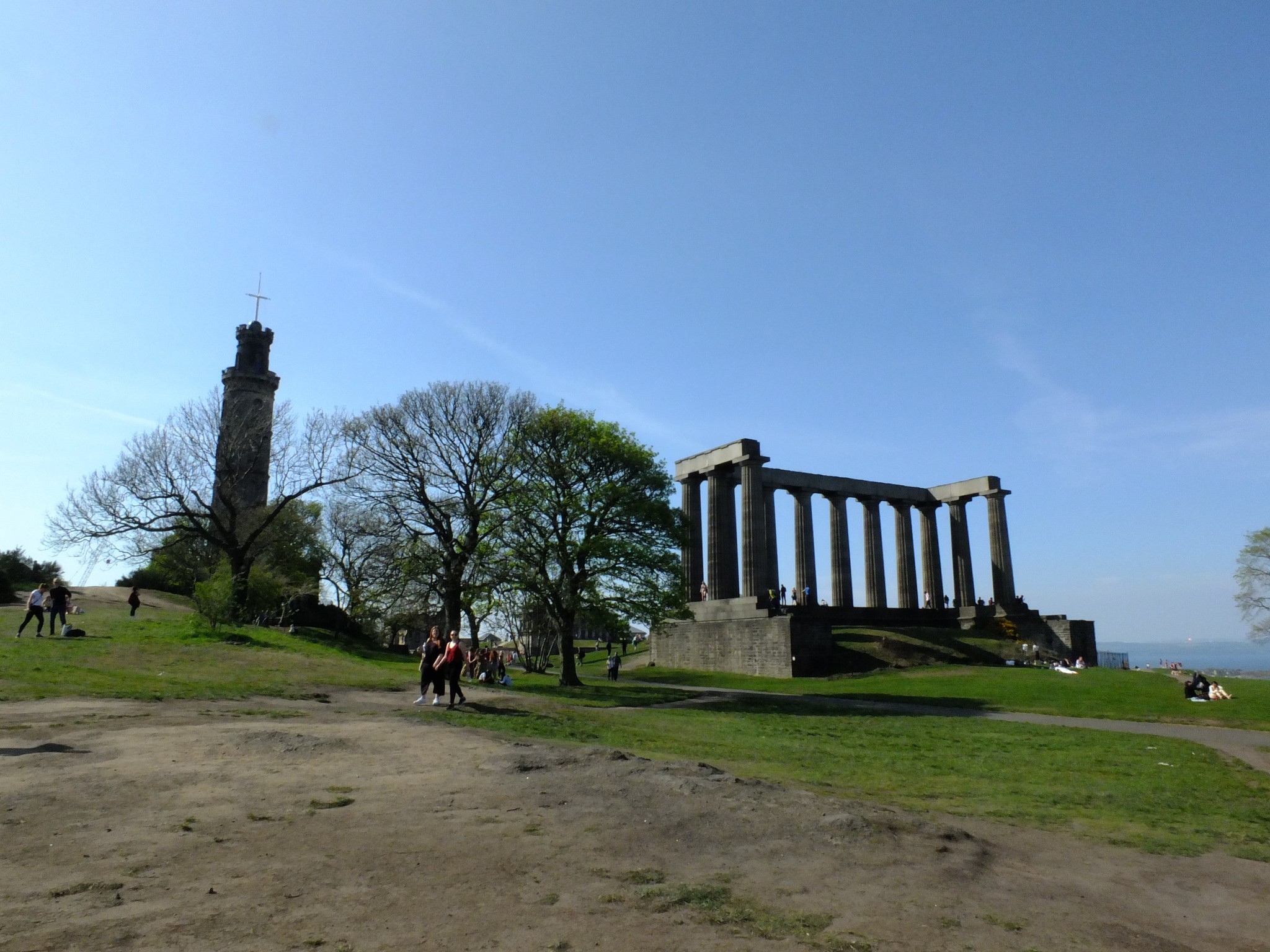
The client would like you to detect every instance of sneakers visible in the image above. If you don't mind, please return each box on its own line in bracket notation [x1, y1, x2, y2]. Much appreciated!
[413, 695, 427, 704]
[433, 697, 439, 705]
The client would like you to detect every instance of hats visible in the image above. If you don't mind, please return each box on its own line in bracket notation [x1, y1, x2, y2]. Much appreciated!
[480, 649, 484, 652]
[492, 648, 495, 651]
[133, 587, 138, 591]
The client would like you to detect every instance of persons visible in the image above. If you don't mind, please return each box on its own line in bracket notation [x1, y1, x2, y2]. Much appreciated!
[1135, 658, 1231, 702]
[128, 587, 140, 616]
[1021, 641, 1086, 675]
[944, 594, 1024, 608]
[924, 591, 932, 608]
[16, 577, 71, 638]
[412, 626, 517, 710]
[767, 584, 828, 606]
[700, 581, 707, 601]
[577, 634, 639, 682]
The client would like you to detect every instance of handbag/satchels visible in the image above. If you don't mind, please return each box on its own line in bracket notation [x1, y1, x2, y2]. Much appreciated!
[61, 623, 73, 635]
[577, 651, 585, 659]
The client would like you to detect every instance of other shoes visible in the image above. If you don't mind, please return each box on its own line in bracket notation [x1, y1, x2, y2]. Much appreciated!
[447, 703, 455, 710]
[16, 633, 20, 638]
[1228, 694, 1232, 699]
[48, 632, 56, 636]
[1221, 695, 1223, 699]
[458, 696, 466, 705]
[36, 634, 44, 638]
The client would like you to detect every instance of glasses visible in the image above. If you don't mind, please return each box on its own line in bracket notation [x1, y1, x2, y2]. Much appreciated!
[54, 582, 59, 583]
[451, 634, 458, 636]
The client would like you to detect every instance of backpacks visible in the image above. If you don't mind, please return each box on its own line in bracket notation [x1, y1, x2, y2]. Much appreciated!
[71, 606, 79, 614]
[1202, 691, 1210, 700]
[478, 671, 489, 683]
[1061, 658, 1071, 667]
[64, 629, 86, 637]
